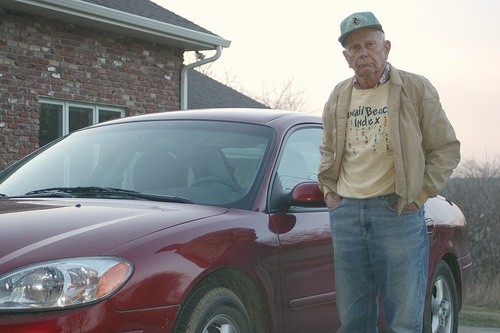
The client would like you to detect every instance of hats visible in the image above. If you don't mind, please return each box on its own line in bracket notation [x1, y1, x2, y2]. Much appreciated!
[338, 11, 382, 43]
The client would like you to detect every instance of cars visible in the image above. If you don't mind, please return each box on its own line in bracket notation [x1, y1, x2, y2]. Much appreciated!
[0, 107, 472, 333]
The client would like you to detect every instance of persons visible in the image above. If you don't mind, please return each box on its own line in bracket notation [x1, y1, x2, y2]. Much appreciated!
[318, 11, 461, 333]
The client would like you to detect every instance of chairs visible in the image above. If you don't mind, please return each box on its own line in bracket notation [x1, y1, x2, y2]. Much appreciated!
[257, 149, 310, 208]
